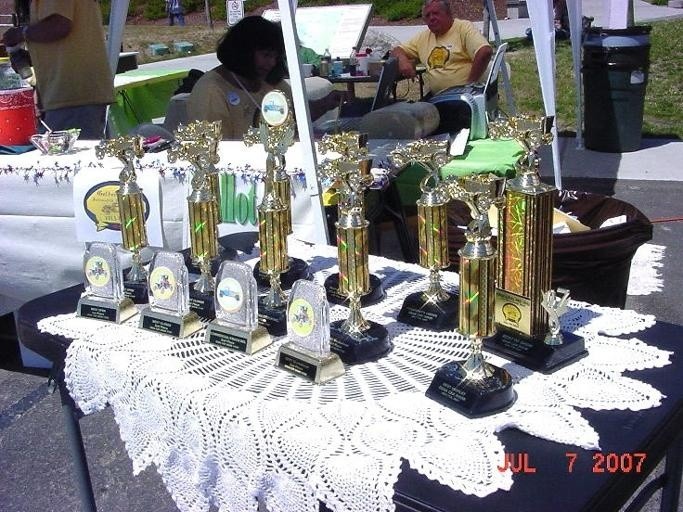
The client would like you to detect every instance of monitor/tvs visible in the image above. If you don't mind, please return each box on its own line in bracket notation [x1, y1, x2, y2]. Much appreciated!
[338, 58, 399, 118]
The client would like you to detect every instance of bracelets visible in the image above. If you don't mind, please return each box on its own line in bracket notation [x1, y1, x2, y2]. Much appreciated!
[21, 25, 29, 41]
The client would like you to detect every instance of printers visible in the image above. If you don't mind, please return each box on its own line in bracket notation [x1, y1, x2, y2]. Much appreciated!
[427, 42, 509, 141]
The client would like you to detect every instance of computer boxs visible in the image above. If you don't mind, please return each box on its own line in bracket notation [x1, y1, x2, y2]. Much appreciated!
[583, 26, 651, 154]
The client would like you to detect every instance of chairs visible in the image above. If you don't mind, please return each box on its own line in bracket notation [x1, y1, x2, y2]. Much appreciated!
[472, 43, 509, 139]
[312, 57, 399, 140]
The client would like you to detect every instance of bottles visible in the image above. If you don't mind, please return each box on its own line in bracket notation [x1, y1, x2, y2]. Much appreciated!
[333, 58, 343, 73]
[323, 48, 330, 63]
[348, 47, 357, 75]
[320, 60, 329, 77]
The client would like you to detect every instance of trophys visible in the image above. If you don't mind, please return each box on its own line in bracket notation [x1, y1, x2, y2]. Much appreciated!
[386, 137, 460, 332]
[94, 134, 157, 305]
[138, 250, 204, 339]
[204, 259, 273, 354]
[242, 89, 314, 335]
[424, 171, 518, 420]
[315, 129, 397, 363]
[166, 118, 224, 321]
[76, 240, 138, 324]
[274, 278, 346, 386]
[480, 108, 590, 375]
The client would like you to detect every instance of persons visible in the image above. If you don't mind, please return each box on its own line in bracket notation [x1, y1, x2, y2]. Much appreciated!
[186, 15, 292, 142]
[2, 0, 118, 141]
[390, 0, 494, 100]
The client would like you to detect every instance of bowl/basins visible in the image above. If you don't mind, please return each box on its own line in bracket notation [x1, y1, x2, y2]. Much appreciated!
[30, 132, 79, 154]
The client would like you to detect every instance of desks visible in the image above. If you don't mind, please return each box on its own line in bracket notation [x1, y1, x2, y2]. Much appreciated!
[0, 139, 415, 367]
[112, 53, 221, 125]
[17, 231, 683, 511]
[319, 66, 425, 102]
